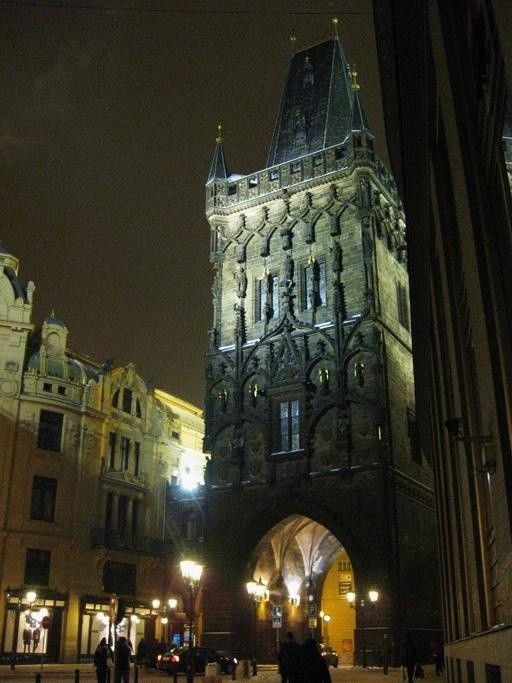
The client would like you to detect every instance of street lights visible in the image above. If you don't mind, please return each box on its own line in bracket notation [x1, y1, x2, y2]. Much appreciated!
[346, 587, 379, 667]
[246, 576, 265, 675]
[153, 597, 177, 642]
[181, 560, 205, 664]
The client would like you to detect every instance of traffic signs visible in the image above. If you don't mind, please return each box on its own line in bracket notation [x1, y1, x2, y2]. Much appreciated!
[272, 606, 282, 629]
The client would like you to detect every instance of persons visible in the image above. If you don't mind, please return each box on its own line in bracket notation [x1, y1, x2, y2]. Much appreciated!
[282, 630, 301, 683]
[278, 641, 287, 674]
[136, 637, 146, 667]
[430, 637, 443, 676]
[22, 623, 33, 653]
[295, 638, 332, 683]
[33, 623, 41, 653]
[403, 653, 416, 682]
[95, 637, 114, 683]
[113, 635, 131, 683]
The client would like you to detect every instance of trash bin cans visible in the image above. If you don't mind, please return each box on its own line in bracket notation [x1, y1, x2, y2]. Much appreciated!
[205, 662, 220, 683]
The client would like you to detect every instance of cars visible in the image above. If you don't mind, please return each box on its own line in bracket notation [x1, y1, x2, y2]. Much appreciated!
[320, 644, 338, 668]
[156, 646, 238, 674]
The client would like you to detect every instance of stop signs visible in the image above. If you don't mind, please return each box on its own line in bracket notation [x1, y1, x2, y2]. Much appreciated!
[42, 617, 50, 629]
